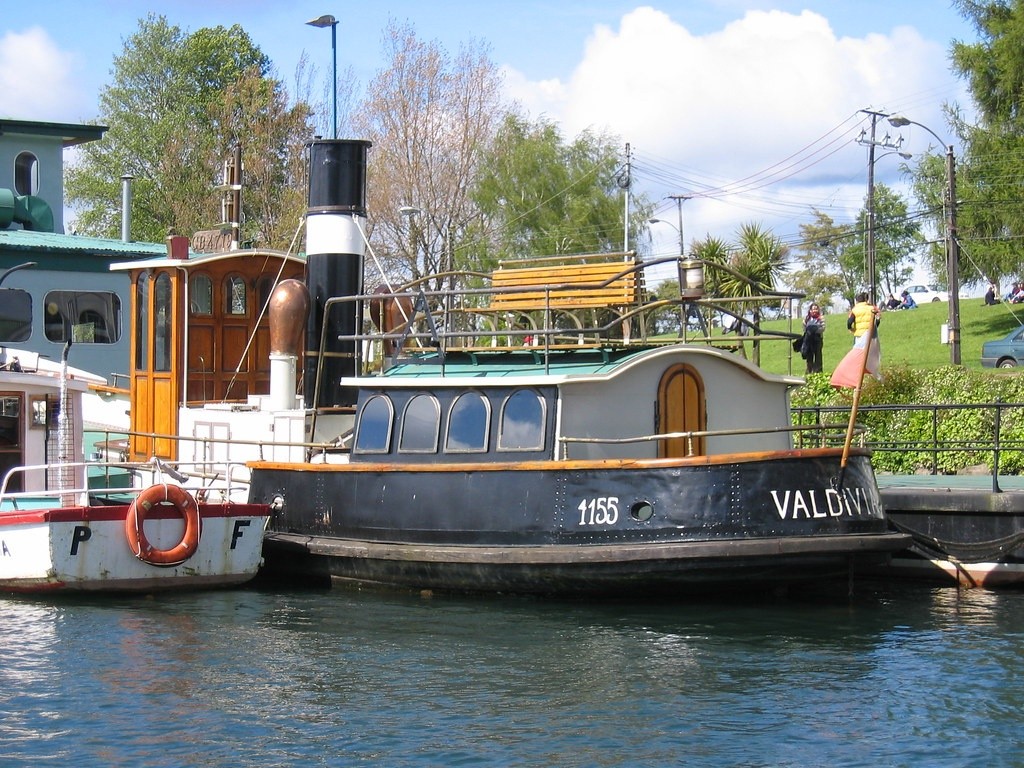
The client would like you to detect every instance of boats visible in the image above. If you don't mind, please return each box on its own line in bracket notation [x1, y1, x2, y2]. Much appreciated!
[0, 16, 913, 593]
[0, 261, 274, 590]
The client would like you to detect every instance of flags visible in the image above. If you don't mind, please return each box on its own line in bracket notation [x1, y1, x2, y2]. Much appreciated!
[827, 316, 883, 393]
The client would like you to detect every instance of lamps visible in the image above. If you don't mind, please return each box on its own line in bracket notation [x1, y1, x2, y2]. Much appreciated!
[678, 258, 709, 298]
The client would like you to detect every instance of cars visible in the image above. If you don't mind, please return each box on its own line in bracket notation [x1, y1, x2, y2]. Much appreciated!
[888, 282, 970, 303]
[978, 324, 1024, 369]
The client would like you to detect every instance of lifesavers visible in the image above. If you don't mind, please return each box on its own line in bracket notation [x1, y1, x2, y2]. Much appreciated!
[125, 483, 200, 566]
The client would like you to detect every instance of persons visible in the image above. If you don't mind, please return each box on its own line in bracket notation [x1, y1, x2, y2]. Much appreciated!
[803, 304, 826, 375]
[879, 290, 918, 312]
[985, 281, 1024, 306]
[846, 292, 881, 347]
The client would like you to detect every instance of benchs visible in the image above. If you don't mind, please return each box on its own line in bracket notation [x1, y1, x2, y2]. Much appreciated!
[497, 250, 658, 341]
[464, 252, 637, 342]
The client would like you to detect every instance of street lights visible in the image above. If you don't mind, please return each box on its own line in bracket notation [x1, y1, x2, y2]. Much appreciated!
[649, 219, 685, 337]
[888, 113, 961, 368]
[305, 15, 341, 141]
